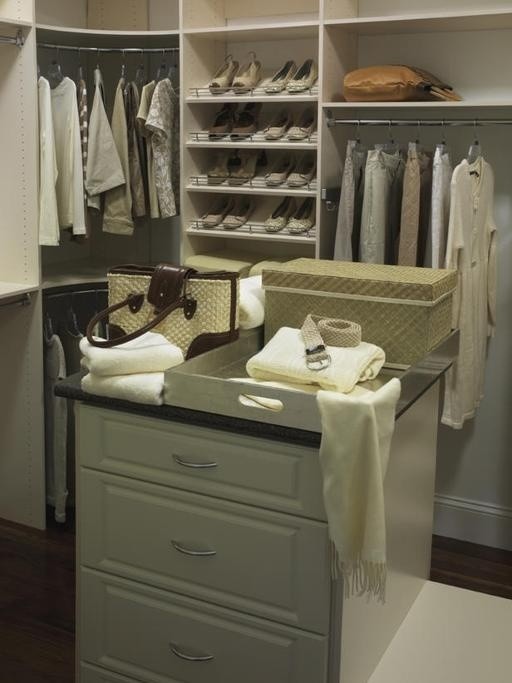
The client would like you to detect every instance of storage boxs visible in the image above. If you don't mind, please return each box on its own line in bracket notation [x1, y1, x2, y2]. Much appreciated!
[262, 256, 458, 373]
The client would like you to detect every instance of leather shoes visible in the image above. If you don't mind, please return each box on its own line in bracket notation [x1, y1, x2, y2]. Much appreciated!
[288, 197, 316, 234]
[203, 196, 234, 228]
[265, 150, 296, 185]
[265, 61, 298, 93]
[287, 106, 318, 140]
[287, 150, 317, 188]
[287, 59, 318, 93]
[264, 197, 296, 232]
[223, 197, 255, 227]
[264, 107, 293, 139]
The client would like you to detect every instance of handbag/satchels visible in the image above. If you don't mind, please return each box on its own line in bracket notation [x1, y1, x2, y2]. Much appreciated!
[86, 264, 240, 359]
[344, 64, 464, 102]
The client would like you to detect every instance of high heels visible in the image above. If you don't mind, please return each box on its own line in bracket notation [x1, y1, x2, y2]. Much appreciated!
[210, 53, 239, 94]
[232, 51, 262, 94]
[208, 103, 238, 139]
[230, 103, 262, 141]
[228, 148, 262, 187]
[208, 148, 236, 186]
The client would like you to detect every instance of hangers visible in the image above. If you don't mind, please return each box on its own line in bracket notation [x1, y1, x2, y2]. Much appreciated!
[37, 43, 180, 90]
[353, 119, 486, 164]
[43, 288, 99, 338]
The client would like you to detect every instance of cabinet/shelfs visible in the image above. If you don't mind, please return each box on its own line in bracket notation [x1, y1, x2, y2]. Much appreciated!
[0, 0, 40, 534]
[322, 0, 512, 551]
[75, 401, 332, 683]
[181, 0, 323, 280]
[40, 0, 180, 534]
